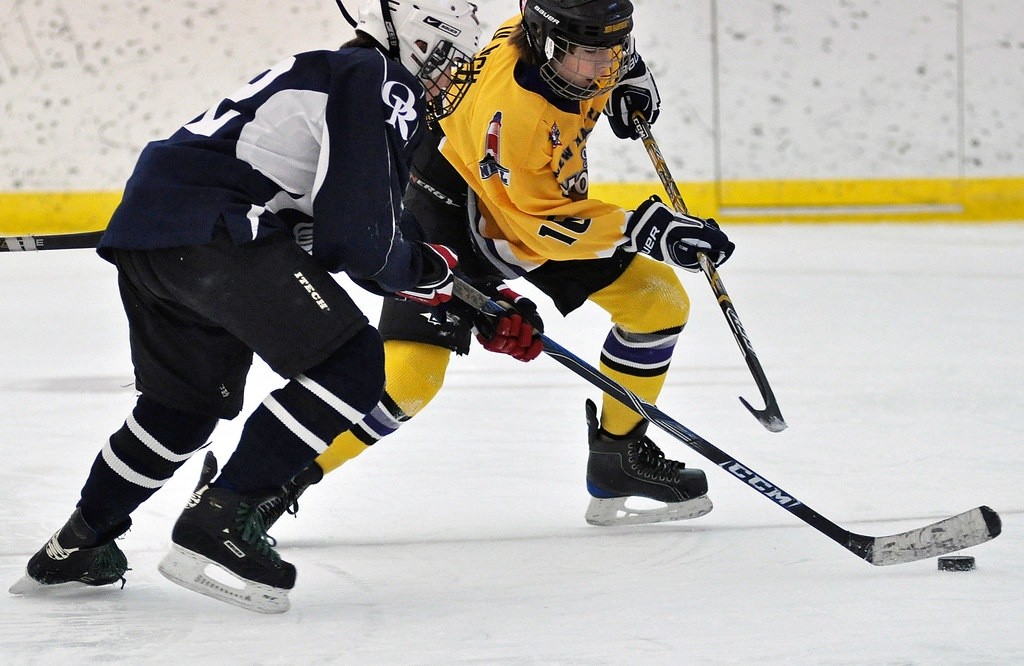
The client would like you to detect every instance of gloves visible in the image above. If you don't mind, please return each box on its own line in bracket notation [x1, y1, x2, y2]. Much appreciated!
[394, 239, 470, 307]
[621, 194, 735, 273]
[470, 277, 545, 363]
[602, 50, 662, 140]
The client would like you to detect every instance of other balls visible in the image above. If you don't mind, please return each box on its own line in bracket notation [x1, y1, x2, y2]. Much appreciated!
[937, 555, 976, 572]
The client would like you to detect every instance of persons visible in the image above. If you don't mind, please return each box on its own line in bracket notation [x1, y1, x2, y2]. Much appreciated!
[263, 0, 734, 539]
[10, 0, 545, 616]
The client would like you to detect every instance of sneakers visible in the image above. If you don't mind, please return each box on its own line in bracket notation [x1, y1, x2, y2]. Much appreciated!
[158, 450, 298, 615]
[7, 505, 134, 596]
[584, 398, 713, 526]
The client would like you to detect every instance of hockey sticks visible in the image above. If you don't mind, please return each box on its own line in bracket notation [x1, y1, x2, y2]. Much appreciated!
[632, 107, 789, 433]
[0, 229, 106, 253]
[393, 207, 1002, 568]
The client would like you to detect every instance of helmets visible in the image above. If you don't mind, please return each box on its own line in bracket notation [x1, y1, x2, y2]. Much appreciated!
[354, 0, 482, 124]
[519, 0, 634, 101]
